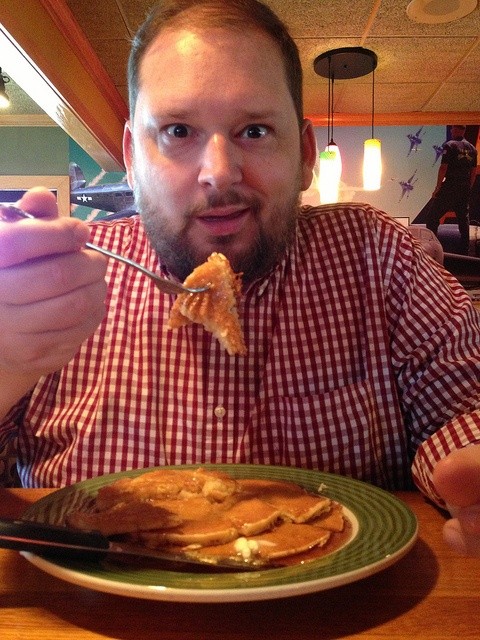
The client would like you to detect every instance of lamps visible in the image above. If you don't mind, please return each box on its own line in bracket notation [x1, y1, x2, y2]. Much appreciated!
[325, 141, 342, 178]
[319, 147, 338, 206]
[0, 68, 10, 109]
[362, 136, 382, 192]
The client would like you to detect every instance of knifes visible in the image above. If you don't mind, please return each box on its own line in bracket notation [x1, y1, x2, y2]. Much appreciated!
[0, 521, 271, 573]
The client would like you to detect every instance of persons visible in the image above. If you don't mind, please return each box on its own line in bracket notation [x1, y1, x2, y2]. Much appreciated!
[0, 2, 478, 555]
[424, 121, 476, 254]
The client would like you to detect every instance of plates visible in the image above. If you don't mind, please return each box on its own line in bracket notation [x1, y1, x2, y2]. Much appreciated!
[20, 462, 418, 604]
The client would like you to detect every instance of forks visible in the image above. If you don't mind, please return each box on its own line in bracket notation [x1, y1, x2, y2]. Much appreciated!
[8, 203, 210, 296]
